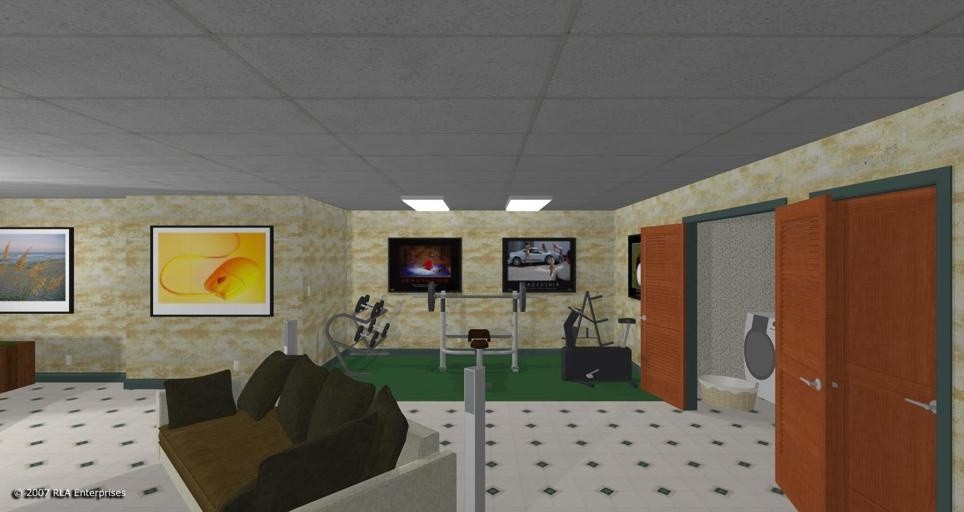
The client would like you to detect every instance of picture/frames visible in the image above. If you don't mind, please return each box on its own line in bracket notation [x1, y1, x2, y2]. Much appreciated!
[150, 224, 276, 318]
[1, 226, 76, 314]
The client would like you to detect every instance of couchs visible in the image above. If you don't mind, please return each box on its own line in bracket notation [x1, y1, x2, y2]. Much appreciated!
[156, 375, 458, 512]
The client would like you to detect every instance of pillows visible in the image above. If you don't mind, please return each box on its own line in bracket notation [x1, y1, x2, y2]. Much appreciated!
[165, 350, 409, 512]
